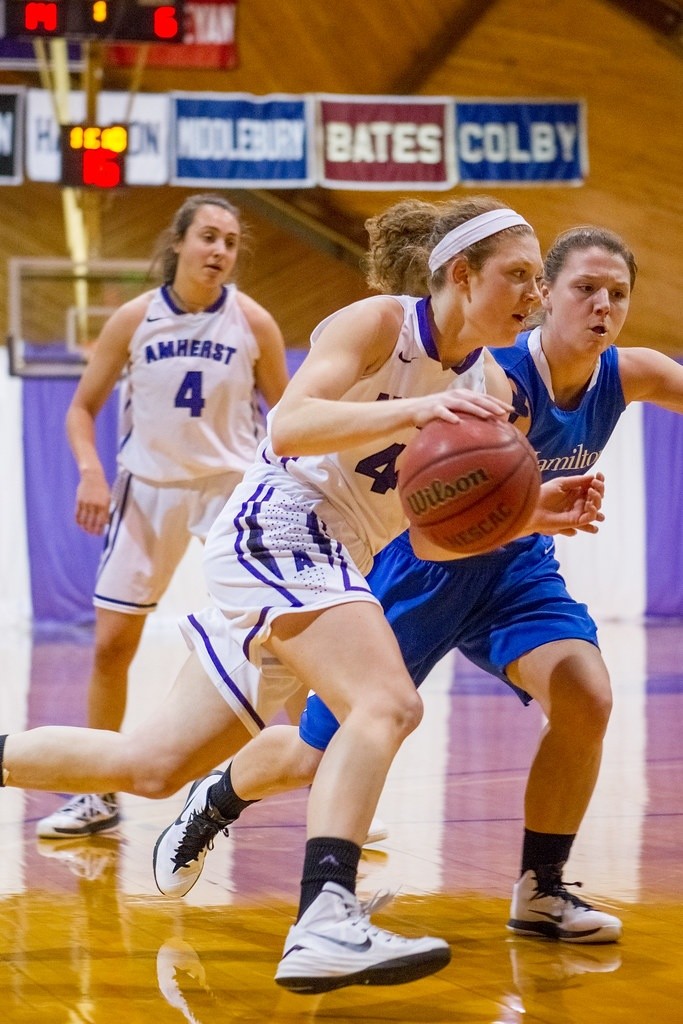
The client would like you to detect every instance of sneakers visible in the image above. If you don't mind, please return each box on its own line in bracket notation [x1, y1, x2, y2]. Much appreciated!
[362, 819, 388, 845]
[275, 881, 453, 993]
[37, 832, 124, 880]
[505, 869, 624, 943]
[156, 940, 229, 1023]
[36, 792, 121, 837]
[508, 937, 621, 987]
[152, 770, 240, 899]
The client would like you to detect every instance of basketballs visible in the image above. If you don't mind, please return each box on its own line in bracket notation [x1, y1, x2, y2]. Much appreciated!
[397, 408, 542, 554]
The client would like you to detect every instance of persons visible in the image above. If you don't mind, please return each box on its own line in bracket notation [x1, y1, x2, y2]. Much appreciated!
[1, 191, 543, 995]
[150, 225, 682, 945]
[33, 198, 389, 846]
